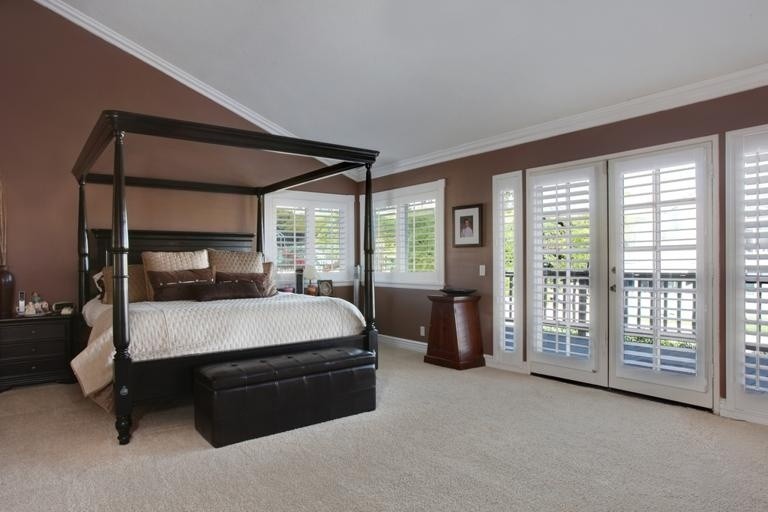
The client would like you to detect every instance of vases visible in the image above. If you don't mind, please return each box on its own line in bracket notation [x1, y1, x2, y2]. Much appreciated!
[0, 265, 15, 319]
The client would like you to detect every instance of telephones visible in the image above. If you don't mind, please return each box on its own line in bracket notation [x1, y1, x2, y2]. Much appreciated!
[15, 290, 26, 316]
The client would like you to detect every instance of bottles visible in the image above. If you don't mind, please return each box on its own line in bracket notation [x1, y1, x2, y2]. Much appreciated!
[295, 269, 304, 294]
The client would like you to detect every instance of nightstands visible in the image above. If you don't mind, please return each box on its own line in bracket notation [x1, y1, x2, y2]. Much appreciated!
[0, 315, 79, 392]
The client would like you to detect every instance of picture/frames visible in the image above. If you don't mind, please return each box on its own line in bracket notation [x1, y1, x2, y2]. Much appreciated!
[452, 203, 484, 248]
[317, 280, 333, 297]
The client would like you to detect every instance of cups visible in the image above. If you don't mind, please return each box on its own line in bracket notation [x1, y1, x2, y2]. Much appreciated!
[27, 302, 49, 313]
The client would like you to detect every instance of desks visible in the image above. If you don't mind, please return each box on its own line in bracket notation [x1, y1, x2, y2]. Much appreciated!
[424, 294, 485, 370]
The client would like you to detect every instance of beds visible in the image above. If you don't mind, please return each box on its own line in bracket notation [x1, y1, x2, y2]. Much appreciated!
[71, 110, 380, 445]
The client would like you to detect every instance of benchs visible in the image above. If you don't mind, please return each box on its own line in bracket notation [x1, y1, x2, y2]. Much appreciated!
[193, 345, 376, 448]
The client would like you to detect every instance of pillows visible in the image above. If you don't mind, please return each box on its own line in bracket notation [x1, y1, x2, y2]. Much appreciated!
[93, 247, 279, 304]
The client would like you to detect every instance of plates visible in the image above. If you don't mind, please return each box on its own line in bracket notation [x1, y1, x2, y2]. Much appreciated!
[19, 311, 52, 317]
[438, 287, 477, 294]
